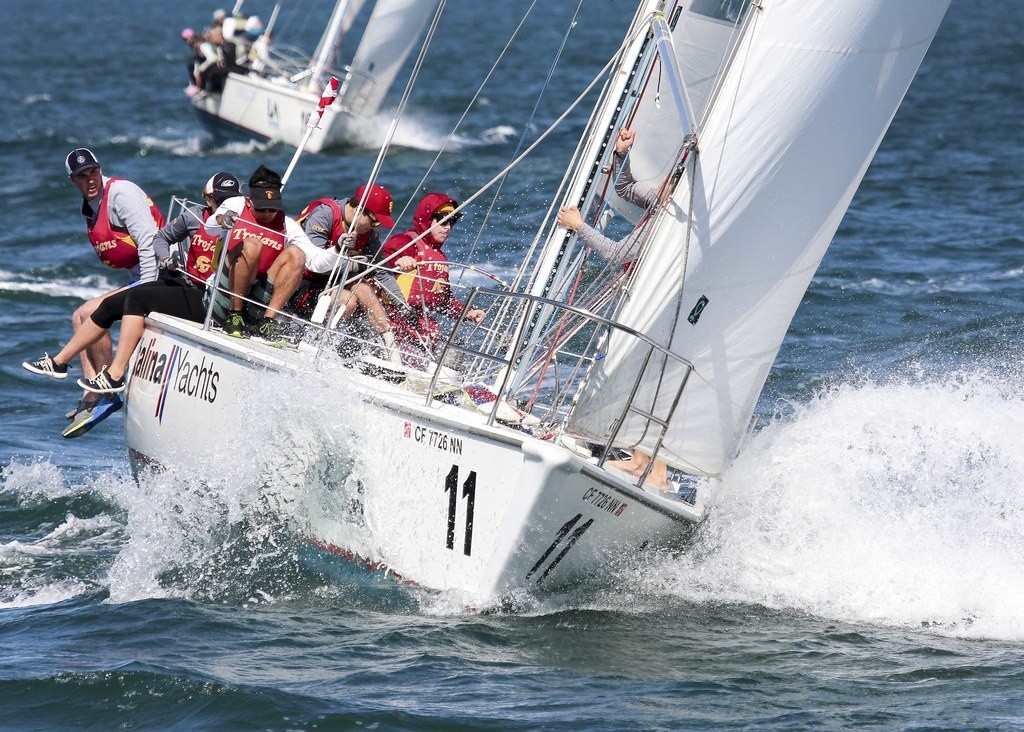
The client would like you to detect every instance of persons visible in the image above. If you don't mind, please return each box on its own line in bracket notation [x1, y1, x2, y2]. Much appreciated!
[206, 166, 358, 347]
[62, 148, 164, 438]
[557, 129, 670, 488]
[23, 171, 243, 393]
[181, 10, 277, 101]
[383, 193, 487, 405]
[294, 183, 416, 383]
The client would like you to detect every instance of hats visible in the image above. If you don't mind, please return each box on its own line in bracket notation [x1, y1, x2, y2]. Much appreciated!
[356, 183, 395, 228]
[66, 148, 100, 177]
[202, 173, 242, 197]
[250, 185, 282, 209]
[435, 202, 456, 212]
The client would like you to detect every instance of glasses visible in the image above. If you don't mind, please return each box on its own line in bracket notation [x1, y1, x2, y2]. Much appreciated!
[209, 196, 223, 207]
[367, 214, 380, 227]
[431, 214, 458, 226]
[254, 208, 278, 213]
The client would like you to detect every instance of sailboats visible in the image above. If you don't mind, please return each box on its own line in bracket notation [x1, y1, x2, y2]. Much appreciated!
[123, 0, 954, 618]
[191, 1, 438, 156]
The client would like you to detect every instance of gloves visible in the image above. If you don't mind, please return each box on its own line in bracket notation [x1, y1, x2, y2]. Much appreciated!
[222, 210, 239, 231]
[161, 257, 175, 271]
[338, 232, 357, 250]
[352, 261, 368, 273]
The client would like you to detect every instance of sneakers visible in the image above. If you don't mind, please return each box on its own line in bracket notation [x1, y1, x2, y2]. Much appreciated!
[220, 314, 251, 339]
[62, 399, 101, 437]
[243, 322, 286, 348]
[76, 365, 126, 393]
[85, 395, 123, 429]
[22, 352, 73, 378]
